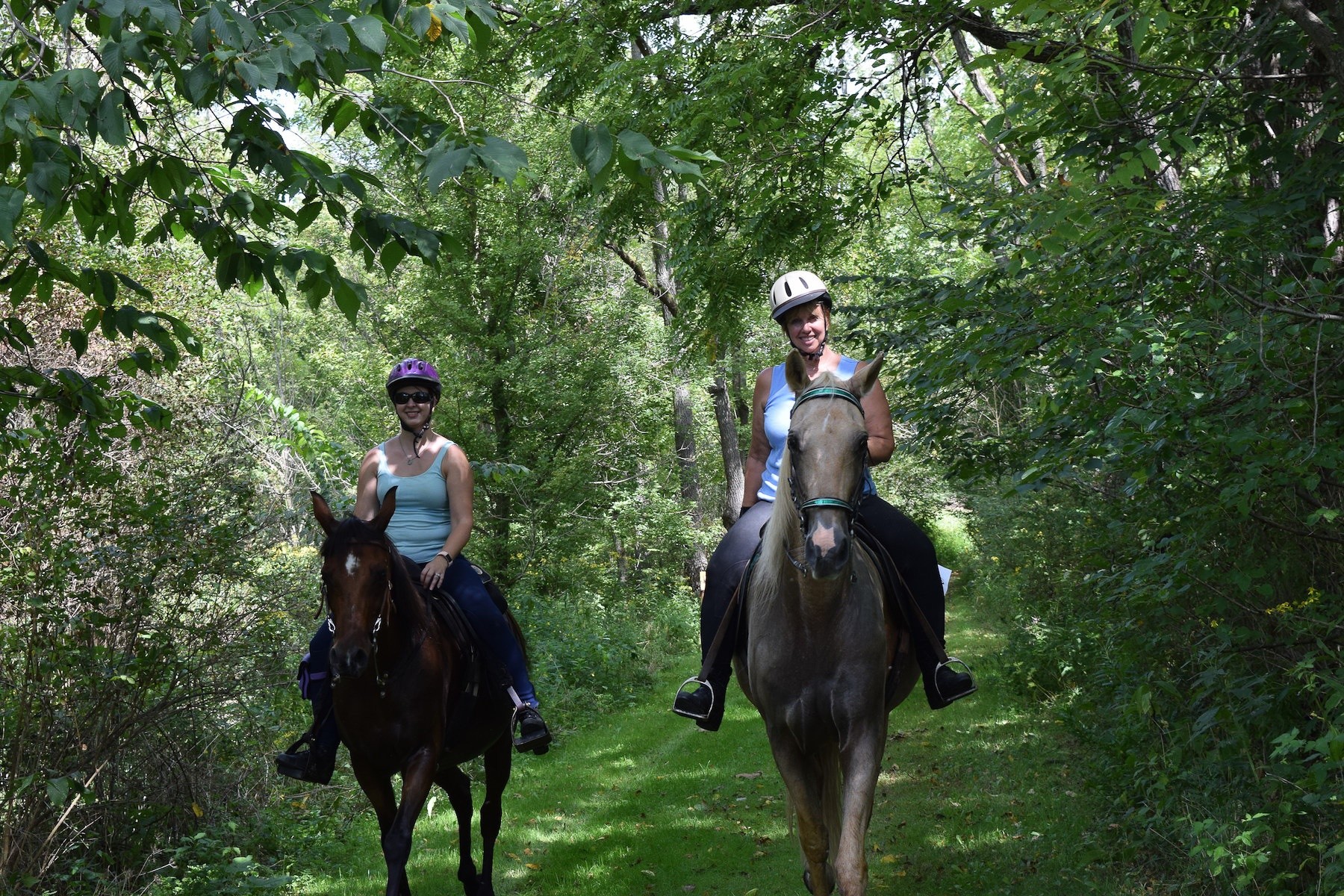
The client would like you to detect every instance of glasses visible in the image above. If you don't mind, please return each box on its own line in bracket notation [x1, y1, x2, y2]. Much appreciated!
[393, 393, 433, 404]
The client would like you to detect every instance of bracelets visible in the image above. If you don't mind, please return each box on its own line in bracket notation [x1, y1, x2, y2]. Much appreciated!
[739, 506, 752, 518]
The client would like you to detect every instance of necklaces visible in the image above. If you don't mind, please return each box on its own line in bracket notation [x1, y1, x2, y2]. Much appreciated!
[398, 431, 434, 465]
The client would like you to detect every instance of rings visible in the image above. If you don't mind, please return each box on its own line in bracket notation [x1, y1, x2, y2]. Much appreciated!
[435, 573, 441, 578]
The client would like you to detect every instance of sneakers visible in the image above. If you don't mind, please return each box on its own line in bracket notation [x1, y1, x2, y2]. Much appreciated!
[679, 686, 727, 731]
[522, 709, 549, 756]
[925, 665, 972, 710]
[274, 744, 335, 785]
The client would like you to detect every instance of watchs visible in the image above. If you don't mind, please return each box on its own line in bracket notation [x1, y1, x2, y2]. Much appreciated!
[436, 551, 453, 567]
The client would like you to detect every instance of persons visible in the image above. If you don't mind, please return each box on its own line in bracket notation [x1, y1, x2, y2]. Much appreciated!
[673, 269, 973, 731]
[276, 357, 551, 786]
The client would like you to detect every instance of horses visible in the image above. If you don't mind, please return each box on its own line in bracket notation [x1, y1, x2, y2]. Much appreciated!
[734, 350, 921, 896]
[311, 485, 538, 896]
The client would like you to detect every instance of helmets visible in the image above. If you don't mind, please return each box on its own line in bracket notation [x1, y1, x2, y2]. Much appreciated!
[768, 270, 832, 321]
[385, 358, 442, 401]
